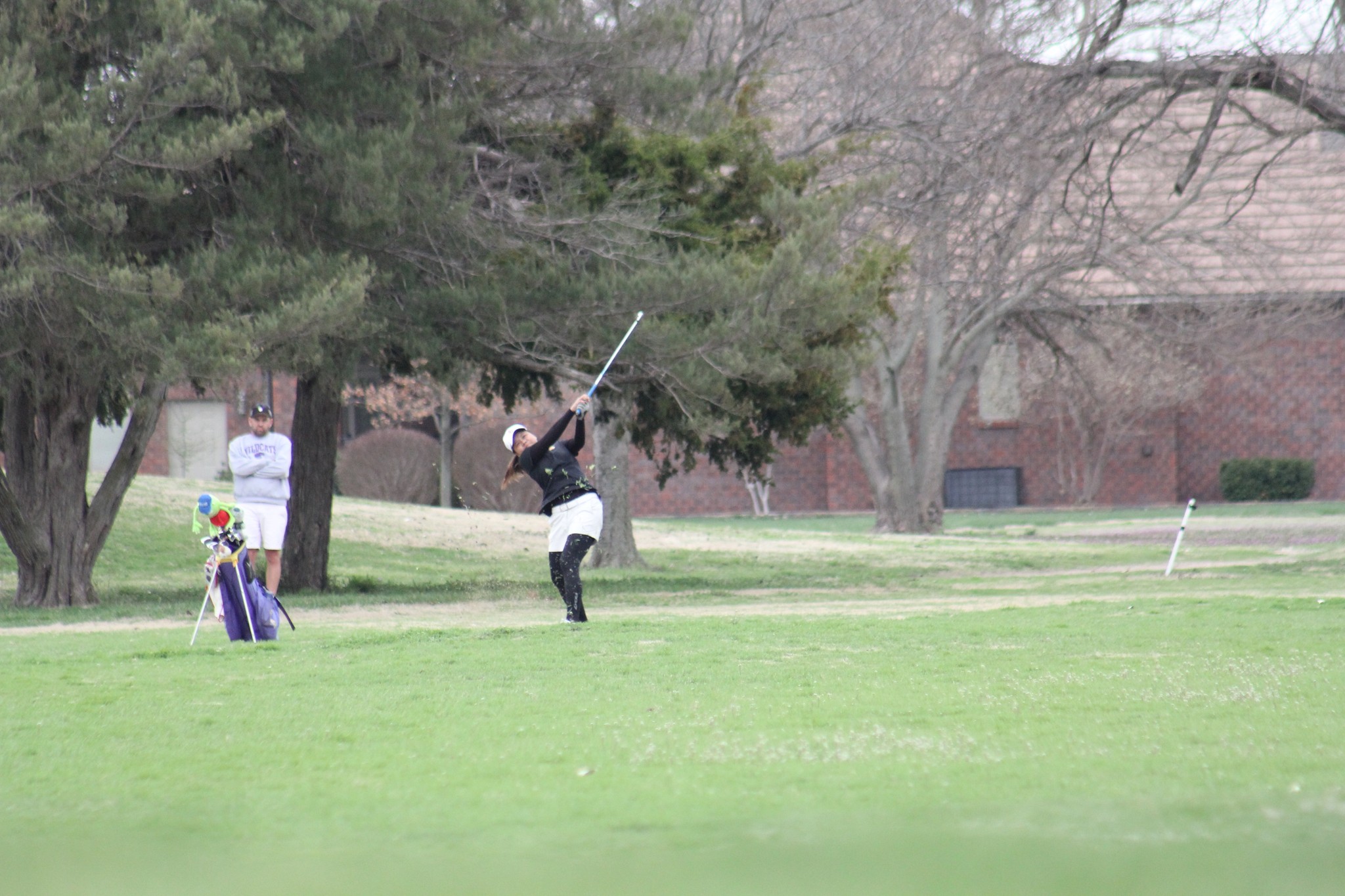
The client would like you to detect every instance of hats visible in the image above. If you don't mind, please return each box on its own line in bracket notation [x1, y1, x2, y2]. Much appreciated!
[249, 405, 272, 419]
[503, 424, 527, 453]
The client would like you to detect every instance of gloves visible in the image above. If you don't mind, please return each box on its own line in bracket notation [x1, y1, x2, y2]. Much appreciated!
[576, 402, 590, 420]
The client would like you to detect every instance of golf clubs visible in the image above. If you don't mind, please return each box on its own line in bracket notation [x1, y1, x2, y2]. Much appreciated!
[575, 310, 645, 416]
[200, 535, 233, 559]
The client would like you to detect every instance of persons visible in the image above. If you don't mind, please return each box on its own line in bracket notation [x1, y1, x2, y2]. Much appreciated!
[228, 403, 292, 597]
[502, 394, 604, 622]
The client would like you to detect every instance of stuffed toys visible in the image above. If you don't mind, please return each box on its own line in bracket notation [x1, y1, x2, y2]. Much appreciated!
[192, 494, 235, 540]
